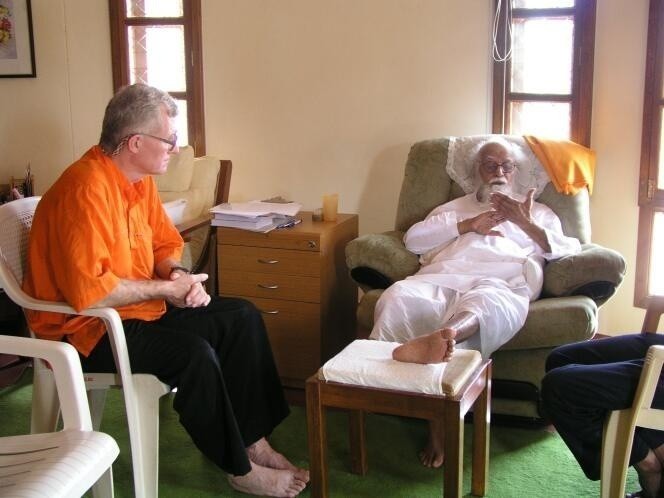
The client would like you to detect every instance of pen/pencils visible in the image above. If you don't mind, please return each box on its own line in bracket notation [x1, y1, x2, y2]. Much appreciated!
[277, 219, 303, 229]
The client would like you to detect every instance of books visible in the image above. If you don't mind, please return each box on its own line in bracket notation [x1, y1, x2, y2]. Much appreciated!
[208, 194, 302, 233]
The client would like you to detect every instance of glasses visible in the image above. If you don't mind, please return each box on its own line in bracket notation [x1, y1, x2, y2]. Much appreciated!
[131, 132, 177, 151]
[480, 160, 517, 173]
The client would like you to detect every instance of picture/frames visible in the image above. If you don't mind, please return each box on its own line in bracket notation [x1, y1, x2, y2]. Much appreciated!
[1, 0, 37, 79]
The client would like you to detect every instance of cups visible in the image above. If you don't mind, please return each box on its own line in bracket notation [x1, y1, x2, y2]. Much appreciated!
[322, 193, 338, 222]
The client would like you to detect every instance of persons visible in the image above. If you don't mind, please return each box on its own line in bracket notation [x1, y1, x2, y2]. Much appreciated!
[366, 135, 582, 471]
[536, 329, 664, 497]
[18, 84, 311, 498]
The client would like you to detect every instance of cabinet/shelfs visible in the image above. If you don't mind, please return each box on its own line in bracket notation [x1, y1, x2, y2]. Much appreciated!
[216, 211, 360, 392]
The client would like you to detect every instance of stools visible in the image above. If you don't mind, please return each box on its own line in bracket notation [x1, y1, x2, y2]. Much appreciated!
[306, 336, 492, 497]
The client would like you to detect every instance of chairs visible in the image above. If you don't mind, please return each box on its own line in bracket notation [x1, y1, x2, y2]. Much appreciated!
[0, 195, 179, 497]
[1, 334, 120, 498]
[345, 136, 625, 431]
[151, 157, 230, 299]
